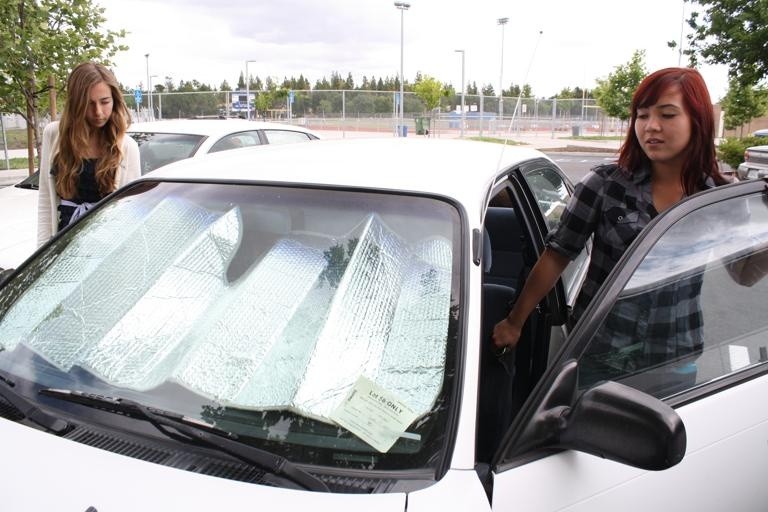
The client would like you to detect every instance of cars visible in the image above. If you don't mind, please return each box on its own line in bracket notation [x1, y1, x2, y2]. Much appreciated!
[0, 141, 768, 509]
[736, 145, 768, 184]
[754, 129, 768, 137]
[0, 119, 321, 290]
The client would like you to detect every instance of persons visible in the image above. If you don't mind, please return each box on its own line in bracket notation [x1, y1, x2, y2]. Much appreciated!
[492, 67, 768, 400]
[37, 63, 148, 321]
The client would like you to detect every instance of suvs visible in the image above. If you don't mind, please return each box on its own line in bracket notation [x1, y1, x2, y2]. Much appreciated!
[217, 101, 257, 120]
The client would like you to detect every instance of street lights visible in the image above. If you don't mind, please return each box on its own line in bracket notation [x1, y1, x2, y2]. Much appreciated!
[455, 50, 466, 137]
[395, 2, 411, 137]
[498, 17, 510, 118]
[245, 59, 257, 121]
[150, 75, 158, 121]
[145, 53, 150, 121]
